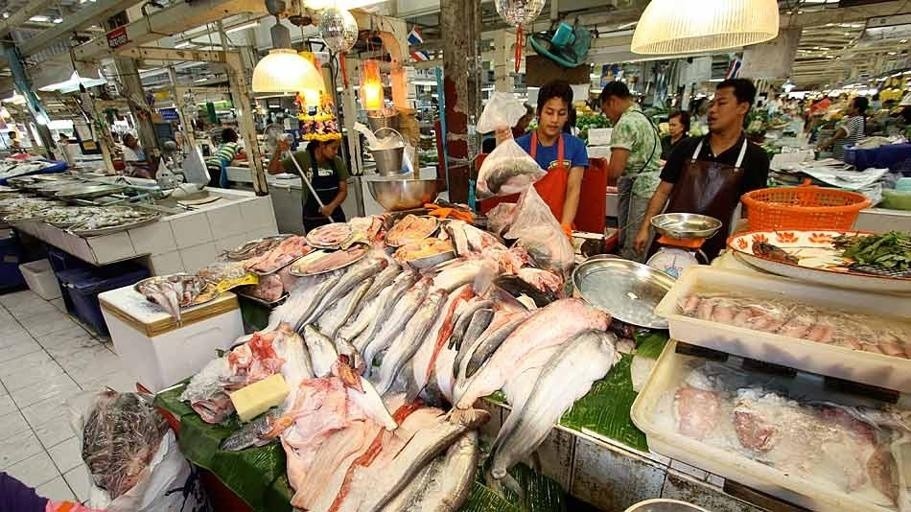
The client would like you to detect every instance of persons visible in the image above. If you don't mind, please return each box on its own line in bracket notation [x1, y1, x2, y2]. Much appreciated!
[643, 83, 711, 160]
[250, 107, 317, 151]
[57, 132, 70, 143]
[633, 79, 769, 264]
[206, 128, 246, 187]
[511, 103, 534, 139]
[104, 110, 138, 146]
[121, 133, 154, 179]
[565, 107, 580, 136]
[598, 81, 670, 263]
[480, 127, 496, 153]
[191, 111, 209, 132]
[497, 79, 589, 239]
[7, 131, 19, 149]
[268, 129, 350, 238]
[767, 85, 911, 149]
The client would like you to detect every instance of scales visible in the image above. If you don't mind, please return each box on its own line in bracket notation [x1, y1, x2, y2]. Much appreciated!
[645, 213, 722, 279]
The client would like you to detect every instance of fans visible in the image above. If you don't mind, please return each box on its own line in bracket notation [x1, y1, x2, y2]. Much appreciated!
[525, 19, 593, 73]
[260, 123, 287, 153]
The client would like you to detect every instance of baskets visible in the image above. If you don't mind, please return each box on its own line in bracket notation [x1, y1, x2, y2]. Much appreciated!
[741, 184, 875, 233]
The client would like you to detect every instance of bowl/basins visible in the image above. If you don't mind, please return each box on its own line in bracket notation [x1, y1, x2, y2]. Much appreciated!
[365, 179, 441, 210]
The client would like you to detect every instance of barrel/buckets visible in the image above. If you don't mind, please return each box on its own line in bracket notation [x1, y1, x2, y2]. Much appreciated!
[368, 127, 405, 175]
[818, 126, 834, 151]
[367, 109, 401, 134]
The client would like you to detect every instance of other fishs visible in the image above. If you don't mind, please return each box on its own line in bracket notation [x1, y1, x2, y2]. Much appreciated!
[81, 222, 639, 512]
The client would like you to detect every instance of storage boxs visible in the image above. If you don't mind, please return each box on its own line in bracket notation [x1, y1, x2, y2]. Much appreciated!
[844, 138, 910, 176]
[96, 272, 246, 394]
[582, 127, 621, 217]
[0, 247, 149, 338]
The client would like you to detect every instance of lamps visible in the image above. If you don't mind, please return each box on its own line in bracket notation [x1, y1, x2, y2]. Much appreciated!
[360, 29, 387, 111]
[247, 2, 324, 100]
[629, 0, 780, 60]
[286, 14, 334, 108]
